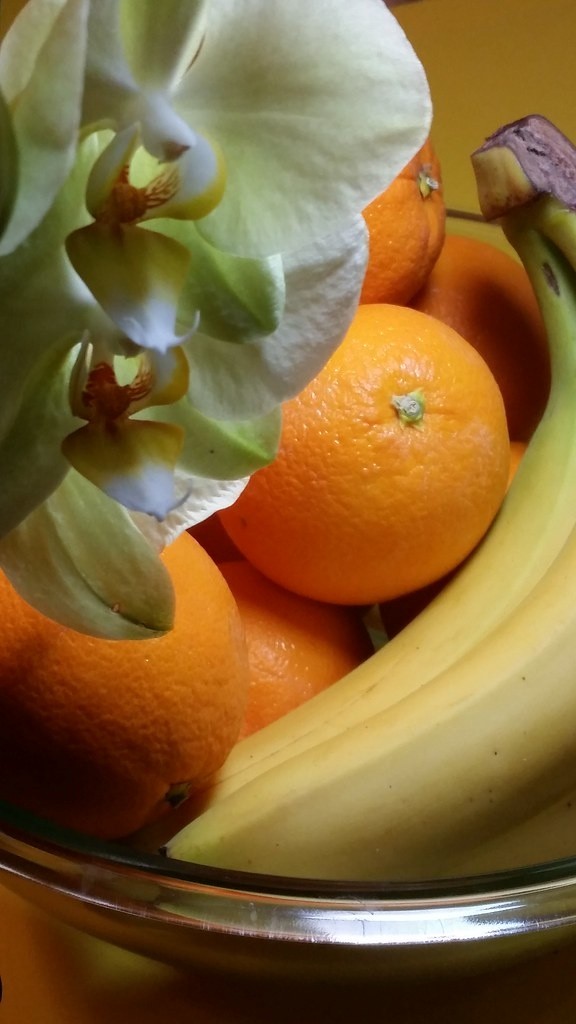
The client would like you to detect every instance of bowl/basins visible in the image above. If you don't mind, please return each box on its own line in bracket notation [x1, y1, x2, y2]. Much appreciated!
[0, 208, 576, 994]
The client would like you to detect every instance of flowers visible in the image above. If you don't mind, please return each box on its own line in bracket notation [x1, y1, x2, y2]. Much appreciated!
[0, 0, 435, 640]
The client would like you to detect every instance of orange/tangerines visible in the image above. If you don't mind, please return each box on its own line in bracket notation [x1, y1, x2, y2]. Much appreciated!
[0, 121, 548, 847]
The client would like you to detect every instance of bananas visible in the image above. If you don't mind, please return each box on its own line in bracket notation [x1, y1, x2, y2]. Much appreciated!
[162, 114, 576, 966]
